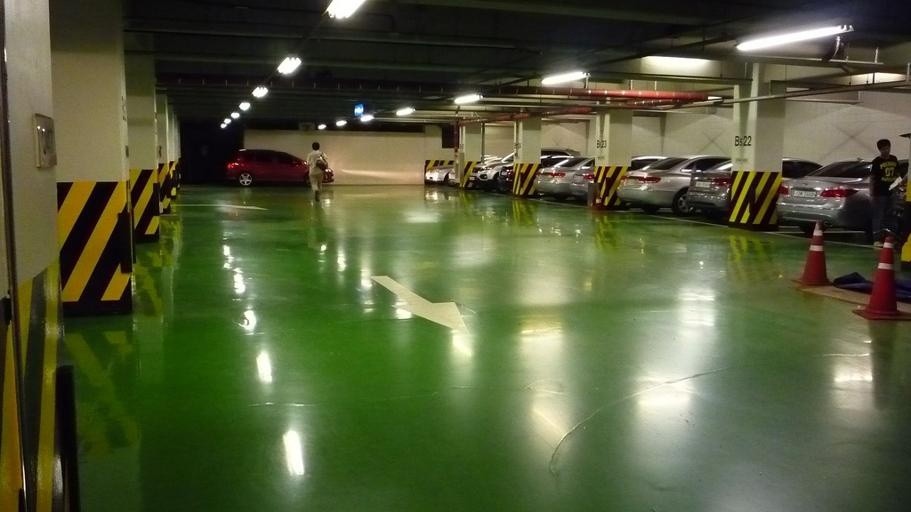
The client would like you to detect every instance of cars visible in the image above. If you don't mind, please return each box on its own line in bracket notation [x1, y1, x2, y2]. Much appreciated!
[617, 155, 731, 217]
[778, 159, 908, 242]
[686, 157, 822, 224]
[222, 148, 334, 187]
[425, 148, 667, 206]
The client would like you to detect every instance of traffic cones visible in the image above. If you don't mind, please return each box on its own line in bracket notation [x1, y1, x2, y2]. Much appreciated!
[852, 230, 911, 320]
[791, 219, 834, 286]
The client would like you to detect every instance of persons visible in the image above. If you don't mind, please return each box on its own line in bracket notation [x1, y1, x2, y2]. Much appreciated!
[895, 173, 911, 251]
[307, 142, 328, 202]
[870, 139, 904, 248]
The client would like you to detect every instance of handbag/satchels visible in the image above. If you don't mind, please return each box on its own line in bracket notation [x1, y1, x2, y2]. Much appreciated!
[316, 151, 329, 171]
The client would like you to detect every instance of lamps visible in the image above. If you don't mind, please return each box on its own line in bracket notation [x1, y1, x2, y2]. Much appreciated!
[395, 69, 590, 117]
[733, 23, 855, 53]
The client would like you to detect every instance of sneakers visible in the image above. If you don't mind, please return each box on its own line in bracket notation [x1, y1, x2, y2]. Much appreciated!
[873, 241, 883, 247]
